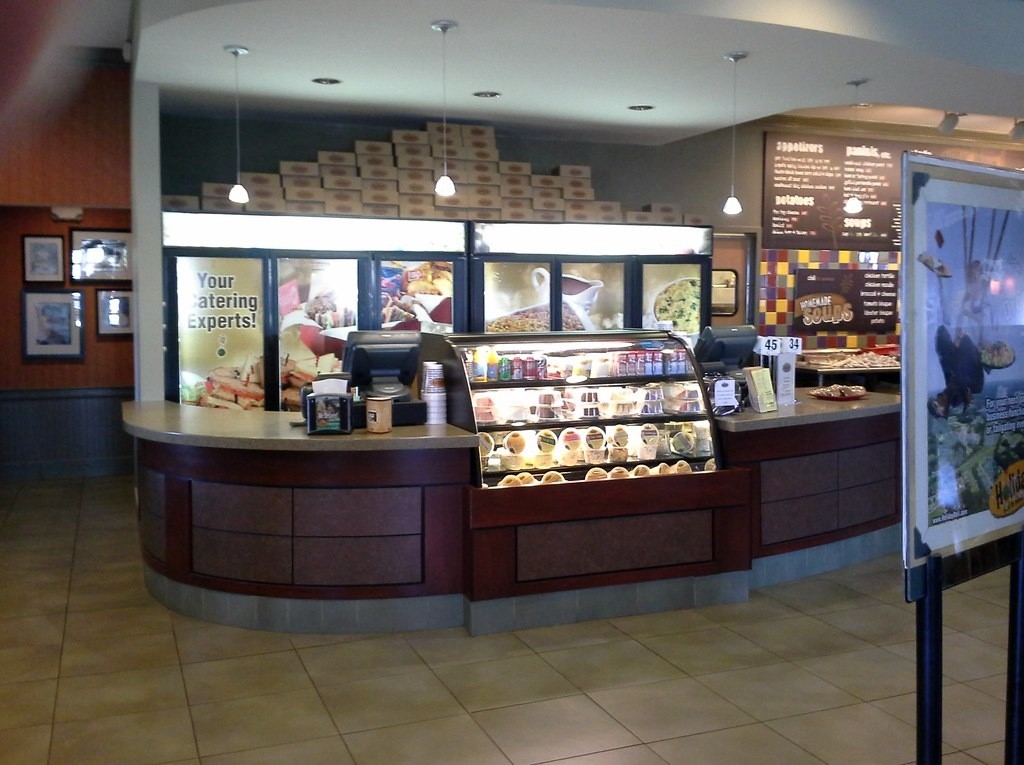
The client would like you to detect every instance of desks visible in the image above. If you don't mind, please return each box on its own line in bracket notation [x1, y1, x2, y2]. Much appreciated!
[796, 362, 901, 387]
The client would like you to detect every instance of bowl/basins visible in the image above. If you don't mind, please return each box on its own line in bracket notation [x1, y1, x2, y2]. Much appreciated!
[652, 278, 700, 335]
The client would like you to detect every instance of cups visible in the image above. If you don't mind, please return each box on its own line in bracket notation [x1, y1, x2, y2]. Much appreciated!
[366, 396, 392, 433]
[421, 361, 447, 424]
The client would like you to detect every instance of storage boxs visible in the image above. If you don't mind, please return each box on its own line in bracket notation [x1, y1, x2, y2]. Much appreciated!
[161, 119, 708, 227]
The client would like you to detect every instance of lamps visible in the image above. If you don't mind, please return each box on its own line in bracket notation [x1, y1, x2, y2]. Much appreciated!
[722, 52, 749, 215]
[223, 46, 249, 204]
[431, 21, 457, 196]
[1007, 117, 1024, 140]
[844, 80, 863, 213]
[936, 111, 967, 135]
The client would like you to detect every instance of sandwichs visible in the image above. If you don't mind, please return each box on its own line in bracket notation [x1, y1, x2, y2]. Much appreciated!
[282, 353, 343, 411]
[196, 354, 266, 411]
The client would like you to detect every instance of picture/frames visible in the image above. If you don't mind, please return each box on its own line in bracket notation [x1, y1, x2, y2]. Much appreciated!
[67, 225, 132, 285]
[95, 287, 134, 341]
[20, 234, 66, 285]
[20, 287, 84, 360]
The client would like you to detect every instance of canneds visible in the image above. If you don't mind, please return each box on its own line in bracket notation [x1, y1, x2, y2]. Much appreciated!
[498, 357, 548, 381]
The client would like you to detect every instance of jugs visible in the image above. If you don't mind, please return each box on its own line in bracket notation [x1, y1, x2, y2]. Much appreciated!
[531, 268, 604, 313]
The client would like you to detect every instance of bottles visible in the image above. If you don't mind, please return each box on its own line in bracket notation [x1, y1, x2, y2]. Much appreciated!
[487, 348, 499, 381]
[474, 347, 486, 381]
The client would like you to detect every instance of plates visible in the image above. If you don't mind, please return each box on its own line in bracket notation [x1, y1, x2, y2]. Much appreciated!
[810, 388, 869, 400]
[486, 300, 595, 332]
[978, 346, 1015, 369]
